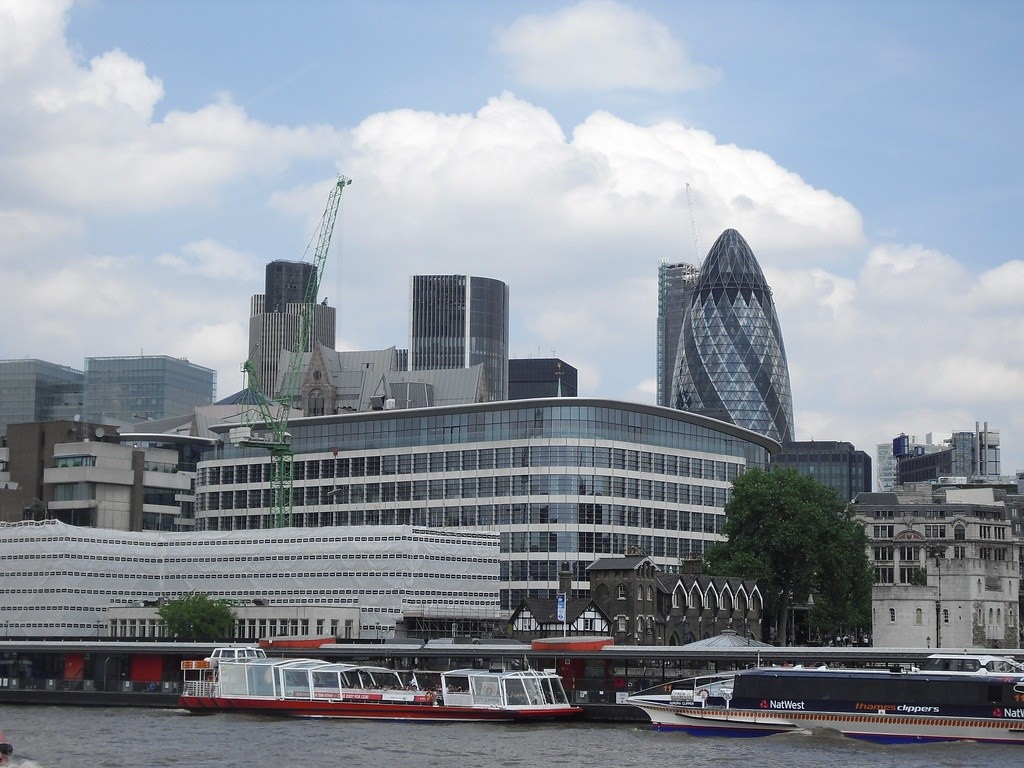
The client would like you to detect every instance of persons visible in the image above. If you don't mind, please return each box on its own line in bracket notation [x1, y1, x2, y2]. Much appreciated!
[829, 635, 871, 647]
[506, 688, 552, 705]
[348, 683, 461, 694]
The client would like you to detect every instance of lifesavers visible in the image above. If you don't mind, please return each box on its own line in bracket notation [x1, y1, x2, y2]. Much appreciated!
[425, 691, 435, 703]
[700, 689, 710, 699]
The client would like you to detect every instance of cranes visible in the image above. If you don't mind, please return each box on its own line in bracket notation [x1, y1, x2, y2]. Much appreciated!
[230, 171, 353, 528]
[684, 182, 703, 265]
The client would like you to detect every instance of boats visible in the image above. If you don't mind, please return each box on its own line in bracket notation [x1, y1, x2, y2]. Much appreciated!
[625, 654, 1024, 745]
[176, 646, 584, 723]
